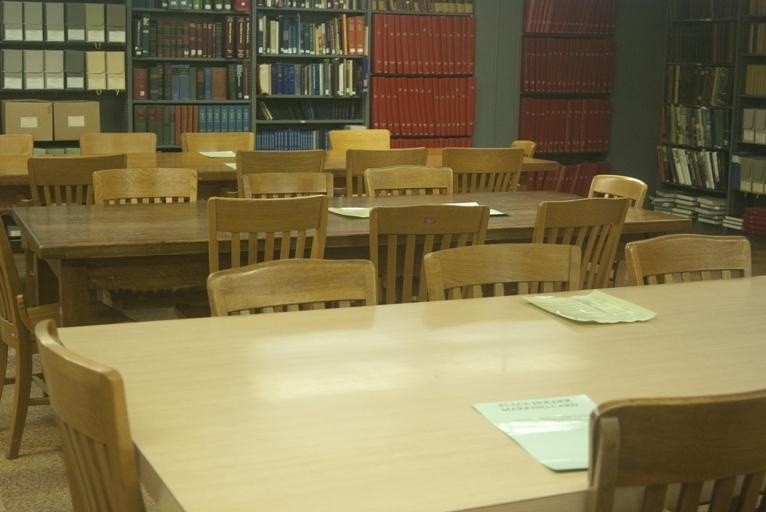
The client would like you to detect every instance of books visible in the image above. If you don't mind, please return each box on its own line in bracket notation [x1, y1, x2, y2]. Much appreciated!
[648, 0, 766, 237]
[133, 1, 252, 147]
[518, 1, 615, 197]
[254, 0, 368, 151]
[371, 1, 475, 150]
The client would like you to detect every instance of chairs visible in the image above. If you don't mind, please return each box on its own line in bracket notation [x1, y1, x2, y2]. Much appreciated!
[625, 233, 753, 285]
[0, 134, 695, 454]
[31, 320, 148, 512]
[586, 386, 765, 512]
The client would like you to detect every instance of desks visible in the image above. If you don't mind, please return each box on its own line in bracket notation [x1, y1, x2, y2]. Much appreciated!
[57, 269, 765, 511]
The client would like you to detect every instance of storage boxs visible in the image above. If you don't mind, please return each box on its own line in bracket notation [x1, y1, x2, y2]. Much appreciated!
[0, 0, 131, 145]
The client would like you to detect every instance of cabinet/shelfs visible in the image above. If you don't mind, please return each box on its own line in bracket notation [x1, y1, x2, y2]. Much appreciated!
[0, 0, 480, 255]
[644, 1, 766, 249]
[498, 1, 616, 201]
